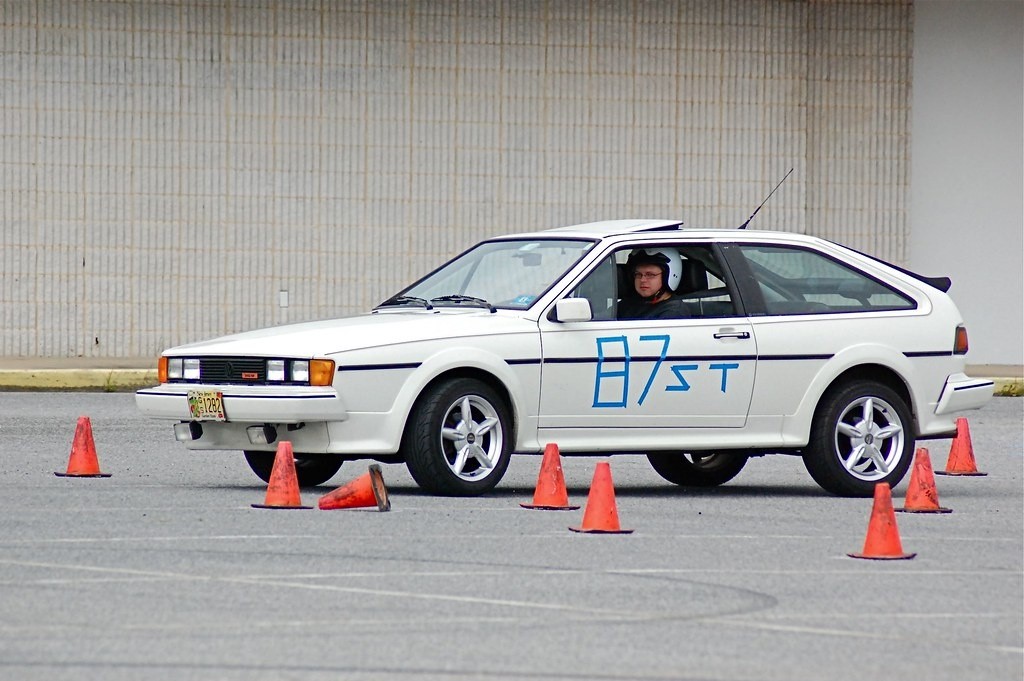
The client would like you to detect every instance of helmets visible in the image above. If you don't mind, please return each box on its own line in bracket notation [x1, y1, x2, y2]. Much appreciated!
[627, 247, 683, 293]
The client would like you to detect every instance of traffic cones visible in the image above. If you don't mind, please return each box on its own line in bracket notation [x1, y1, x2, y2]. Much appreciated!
[251, 441, 315, 510]
[933, 417, 990, 478]
[319, 462, 391, 514]
[53, 415, 116, 477]
[891, 446, 954, 515]
[519, 442, 582, 513]
[847, 482, 918, 562]
[566, 463, 635, 534]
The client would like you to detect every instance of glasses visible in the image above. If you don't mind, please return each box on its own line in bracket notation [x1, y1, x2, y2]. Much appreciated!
[634, 272, 662, 279]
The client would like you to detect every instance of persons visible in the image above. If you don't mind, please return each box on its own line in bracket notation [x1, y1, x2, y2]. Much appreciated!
[594, 246, 692, 321]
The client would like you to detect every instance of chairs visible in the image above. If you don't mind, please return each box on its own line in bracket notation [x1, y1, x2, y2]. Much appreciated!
[597, 263, 629, 300]
[675, 258, 708, 314]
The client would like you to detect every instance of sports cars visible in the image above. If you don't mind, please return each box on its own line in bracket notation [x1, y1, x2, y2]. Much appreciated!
[133, 217, 1000, 495]
